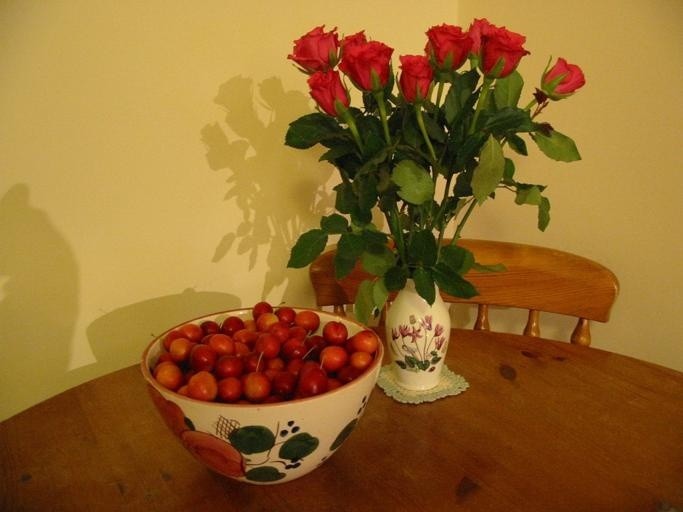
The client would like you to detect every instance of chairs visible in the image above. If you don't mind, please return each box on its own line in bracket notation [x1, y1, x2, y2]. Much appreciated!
[309, 238, 620, 347]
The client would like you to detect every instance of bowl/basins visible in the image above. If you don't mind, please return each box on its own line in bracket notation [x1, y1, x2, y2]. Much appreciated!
[139, 305, 386, 486]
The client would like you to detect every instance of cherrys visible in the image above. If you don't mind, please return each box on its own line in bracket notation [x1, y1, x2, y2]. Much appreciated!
[151, 301, 379, 405]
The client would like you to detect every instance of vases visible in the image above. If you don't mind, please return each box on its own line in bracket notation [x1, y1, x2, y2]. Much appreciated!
[384, 277, 452, 392]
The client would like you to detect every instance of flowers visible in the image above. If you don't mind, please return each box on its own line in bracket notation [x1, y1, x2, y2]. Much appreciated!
[283, 17, 586, 328]
